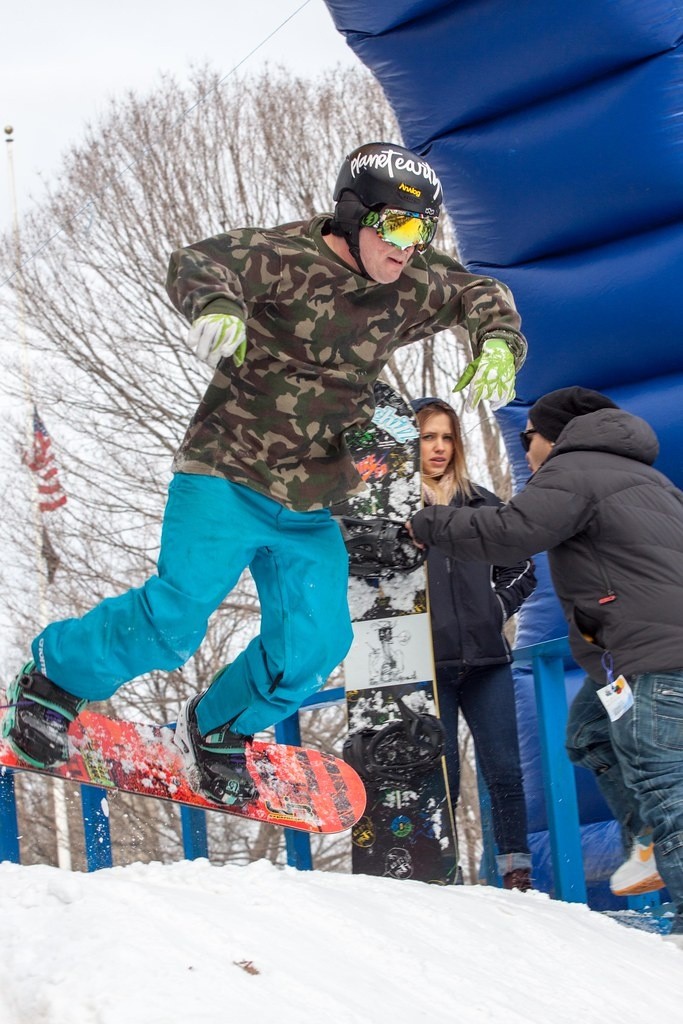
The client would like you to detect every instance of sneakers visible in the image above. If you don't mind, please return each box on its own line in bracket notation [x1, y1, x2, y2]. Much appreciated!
[609, 830, 665, 896]
[174, 663, 261, 808]
[0, 656, 89, 768]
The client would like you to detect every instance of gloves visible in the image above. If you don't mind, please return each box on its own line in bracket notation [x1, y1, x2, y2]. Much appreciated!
[450, 338, 517, 413]
[187, 312, 248, 368]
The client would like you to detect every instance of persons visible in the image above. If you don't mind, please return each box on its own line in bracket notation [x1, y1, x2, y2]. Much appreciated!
[399, 396, 549, 907]
[10, 142, 526, 807]
[404, 386, 682, 932]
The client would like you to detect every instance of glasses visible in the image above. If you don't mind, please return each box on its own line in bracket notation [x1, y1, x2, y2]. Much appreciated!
[361, 208, 440, 255]
[519, 429, 538, 451]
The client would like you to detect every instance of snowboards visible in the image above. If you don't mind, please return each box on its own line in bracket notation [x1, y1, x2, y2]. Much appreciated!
[330, 377, 462, 891]
[1, 681, 367, 833]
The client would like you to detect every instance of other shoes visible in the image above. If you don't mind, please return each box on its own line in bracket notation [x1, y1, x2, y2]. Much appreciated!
[502, 868, 533, 892]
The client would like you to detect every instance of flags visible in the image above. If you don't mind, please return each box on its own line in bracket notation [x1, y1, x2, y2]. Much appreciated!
[21, 405, 66, 584]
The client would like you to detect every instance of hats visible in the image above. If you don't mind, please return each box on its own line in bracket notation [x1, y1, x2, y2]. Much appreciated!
[527, 386, 621, 444]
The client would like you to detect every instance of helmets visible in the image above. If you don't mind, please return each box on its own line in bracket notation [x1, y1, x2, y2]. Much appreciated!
[332, 142, 443, 236]
